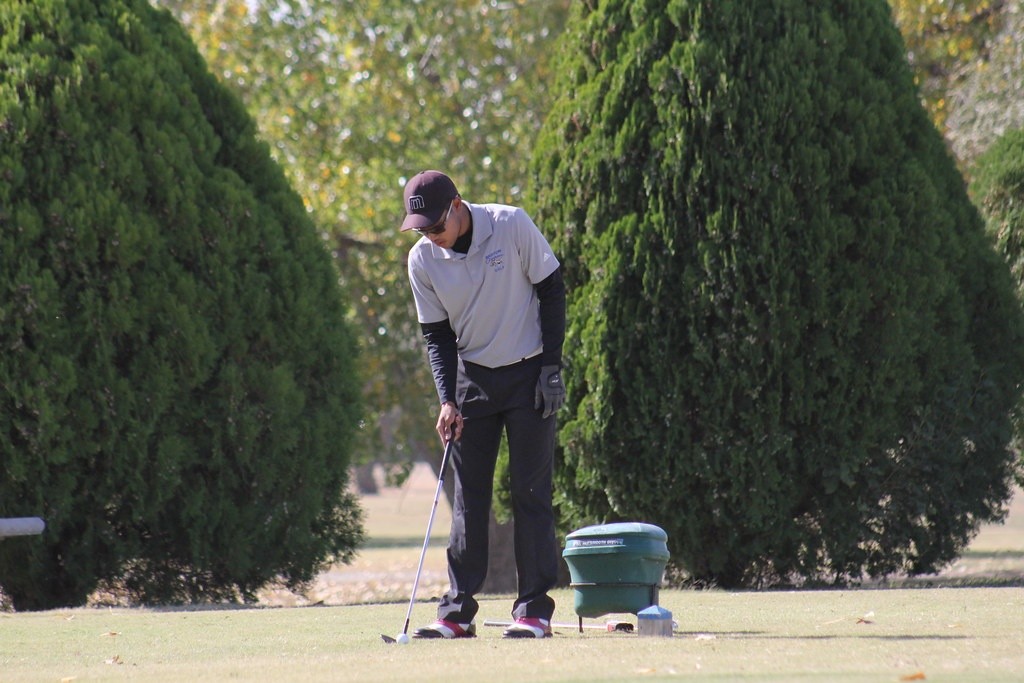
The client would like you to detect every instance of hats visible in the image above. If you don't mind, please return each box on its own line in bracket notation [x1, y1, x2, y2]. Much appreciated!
[401, 171, 458, 232]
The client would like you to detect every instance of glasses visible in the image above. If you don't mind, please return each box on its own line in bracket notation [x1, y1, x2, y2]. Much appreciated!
[413, 200, 454, 236]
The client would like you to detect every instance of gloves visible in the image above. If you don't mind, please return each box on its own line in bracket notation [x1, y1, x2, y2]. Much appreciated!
[534, 364, 565, 418]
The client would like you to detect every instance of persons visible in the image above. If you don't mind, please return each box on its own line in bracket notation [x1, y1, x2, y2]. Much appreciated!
[401, 170, 566, 638]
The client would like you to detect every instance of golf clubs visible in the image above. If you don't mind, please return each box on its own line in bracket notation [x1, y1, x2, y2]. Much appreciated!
[483, 620, 634, 631]
[380, 412, 459, 644]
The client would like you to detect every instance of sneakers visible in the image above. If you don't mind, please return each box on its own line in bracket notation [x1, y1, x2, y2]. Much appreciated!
[502, 617, 553, 639]
[412, 619, 476, 638]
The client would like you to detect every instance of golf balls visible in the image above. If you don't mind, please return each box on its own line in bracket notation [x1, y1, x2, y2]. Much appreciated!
[396, 634, 409, 644]
